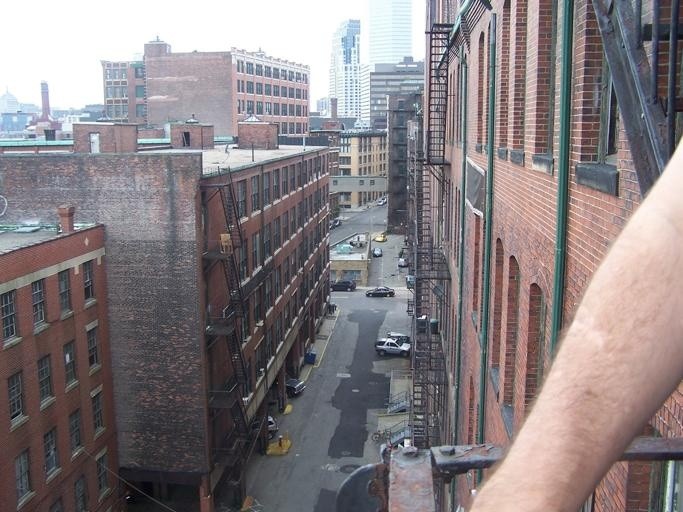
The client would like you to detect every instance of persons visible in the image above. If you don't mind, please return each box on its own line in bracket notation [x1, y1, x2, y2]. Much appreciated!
[468, 134, 682, 511]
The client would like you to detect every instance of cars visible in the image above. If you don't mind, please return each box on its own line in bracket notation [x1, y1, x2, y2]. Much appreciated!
[376, 195, 387, 206]
[374, 234, 388, 242]
[372, 246, 382, 257]
[328, 219, 340, 231]
[365, 285, 396, 298]
[397, 257, 409, 268]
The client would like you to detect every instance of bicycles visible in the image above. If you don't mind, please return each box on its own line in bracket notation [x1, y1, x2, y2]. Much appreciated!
[370, 425, 393, 443]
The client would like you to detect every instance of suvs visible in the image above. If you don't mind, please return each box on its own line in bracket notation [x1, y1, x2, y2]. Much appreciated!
[253, 415, 278, 441]
[330, 279, 356, 292]
[373, 336, 411, 357]
[274, 372, 306, 398]
